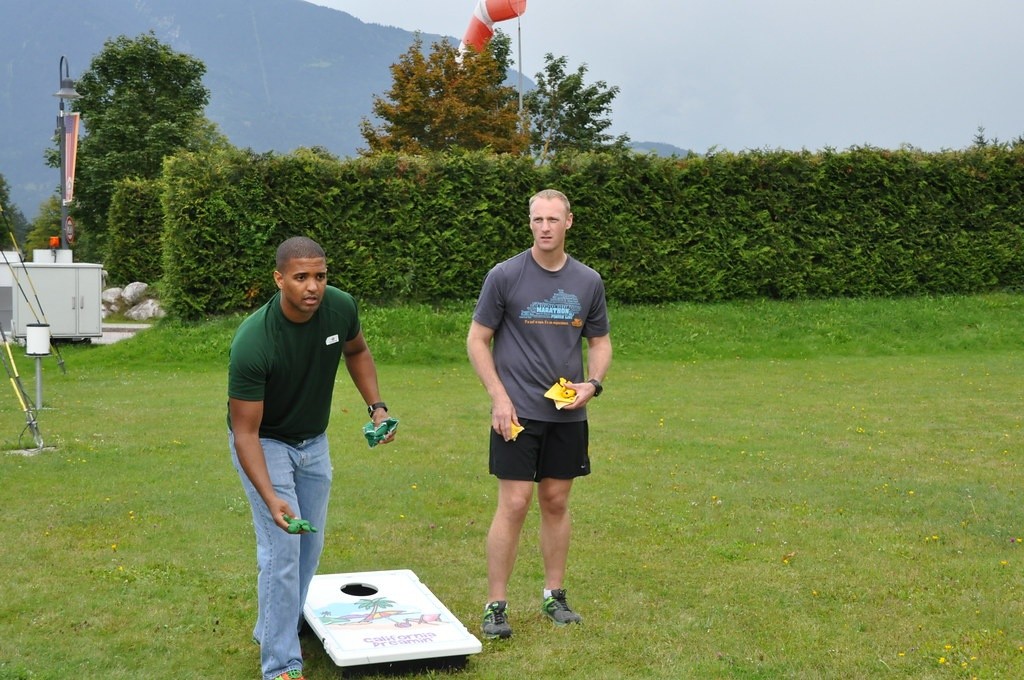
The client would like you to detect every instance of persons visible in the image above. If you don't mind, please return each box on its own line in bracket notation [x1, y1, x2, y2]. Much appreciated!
[466, 188, 613, 639]
[226, 236, 398, 680]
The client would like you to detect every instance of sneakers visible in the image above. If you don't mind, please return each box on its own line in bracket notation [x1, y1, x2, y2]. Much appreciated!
[542, 588, 581, 626]
[480, 600, 512, 639]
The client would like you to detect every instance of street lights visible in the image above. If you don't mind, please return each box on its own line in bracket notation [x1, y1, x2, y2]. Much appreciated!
[52, 55, 85, 250]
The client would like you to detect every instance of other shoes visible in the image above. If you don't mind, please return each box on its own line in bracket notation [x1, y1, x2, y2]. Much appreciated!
[273, 670, 307, 680]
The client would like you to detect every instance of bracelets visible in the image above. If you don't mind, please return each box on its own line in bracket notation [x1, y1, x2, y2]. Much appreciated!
[368, 402, 387, 418]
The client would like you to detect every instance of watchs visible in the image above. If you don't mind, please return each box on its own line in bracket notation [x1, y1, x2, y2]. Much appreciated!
[589, 378, 603, 396]
